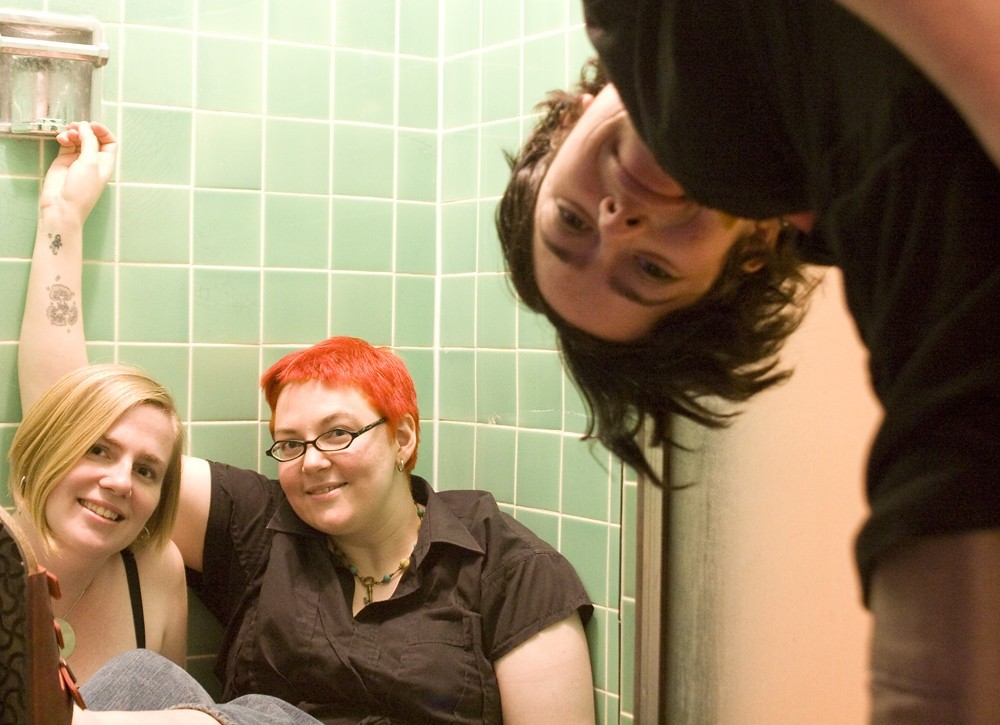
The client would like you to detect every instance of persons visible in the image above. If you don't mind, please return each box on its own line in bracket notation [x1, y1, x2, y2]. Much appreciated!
[1, 121, 596, 725]
[495, 0, 1000, 725]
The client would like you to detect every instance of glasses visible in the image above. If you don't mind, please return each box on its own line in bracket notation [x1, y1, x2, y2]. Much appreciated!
[265, 417, 387, 462]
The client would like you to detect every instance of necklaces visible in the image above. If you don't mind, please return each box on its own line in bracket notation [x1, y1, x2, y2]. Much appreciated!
[327, 499, 427, 608]
[52, 577, 100, 659]
